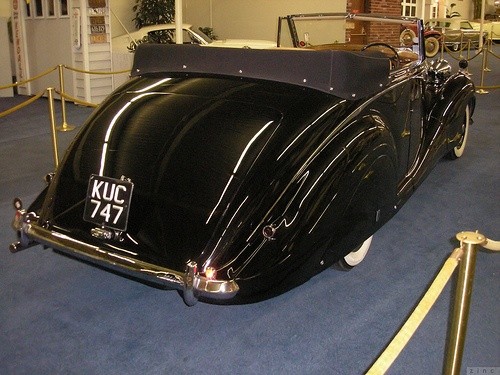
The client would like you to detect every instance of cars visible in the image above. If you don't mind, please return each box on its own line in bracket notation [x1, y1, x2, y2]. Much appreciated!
[7, 12, 477, 306]
[113, 24, 281, 48]
[396, 16, 441, 57]
[425, 18, 488, 52]
